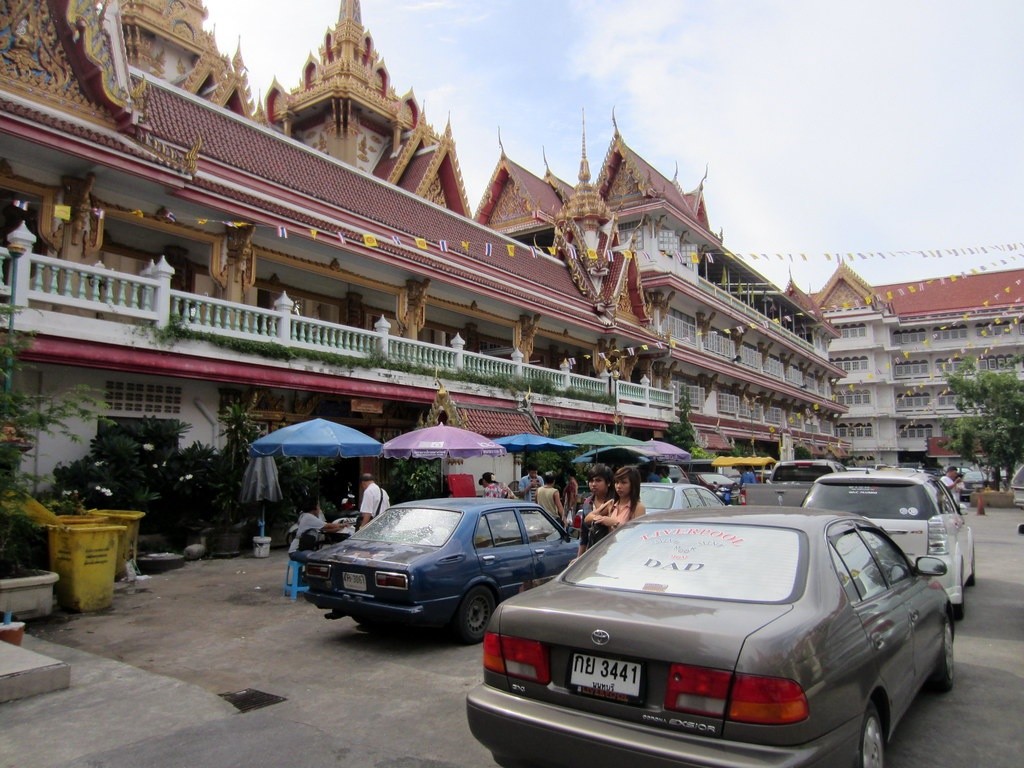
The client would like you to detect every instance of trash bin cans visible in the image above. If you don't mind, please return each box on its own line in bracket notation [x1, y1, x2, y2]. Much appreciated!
[57, 515, 109, 524]
[47, 525, 127, 612]
[88, 509, 146, 582]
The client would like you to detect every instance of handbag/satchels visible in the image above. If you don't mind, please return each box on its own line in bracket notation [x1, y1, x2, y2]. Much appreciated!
[299, 529, 325, 551]
[566, 510, 573, 527]
[355, 516, 372, 532]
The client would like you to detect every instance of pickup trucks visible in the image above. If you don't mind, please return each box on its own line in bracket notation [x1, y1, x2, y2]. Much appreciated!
[739, 459, 848, 508]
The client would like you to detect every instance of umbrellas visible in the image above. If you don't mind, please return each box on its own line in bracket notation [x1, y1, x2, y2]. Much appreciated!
[383, 423, 507, 497]
[555, 428, 691, 466]
[241, 429, 284, 538]
[489, 432, 581, 467]
[248, 418, 385, 498]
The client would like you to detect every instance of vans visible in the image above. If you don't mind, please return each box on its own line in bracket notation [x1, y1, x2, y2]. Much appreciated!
[661, 459, 733, 477]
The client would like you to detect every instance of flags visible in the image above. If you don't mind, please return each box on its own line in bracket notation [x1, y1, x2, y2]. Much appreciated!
[12, 198, 1024, 263]
[568, 253, 1024, 366]
[768, 362, 952, 447]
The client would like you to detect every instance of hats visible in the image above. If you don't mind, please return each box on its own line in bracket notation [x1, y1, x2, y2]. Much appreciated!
[483, 472, 495, 482]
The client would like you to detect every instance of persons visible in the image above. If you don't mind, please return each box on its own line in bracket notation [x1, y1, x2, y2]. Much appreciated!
[534, 475, 568, 532]
[518, 463, 545, 504]
[286, 495, 350, 585]
[654, 465, 673, 483]
[939, 466, 961, 509]
[646, 462, 660, 483]
[584, 466, 646, 533]
[479, 472, 519, 499]
[728, 465, 757, 486]
[358, 472, 391, 530]
[562, 468, 579, 532]
[577, 463, 615, 558]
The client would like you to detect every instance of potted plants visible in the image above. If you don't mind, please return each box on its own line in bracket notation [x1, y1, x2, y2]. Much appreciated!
[187, 397, 335, 556]
[938, 352, 1024, 507]
[0, 306, 119, 621]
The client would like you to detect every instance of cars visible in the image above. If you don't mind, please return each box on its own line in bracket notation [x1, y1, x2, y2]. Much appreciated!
[659, 464, 691, 484]
[685, 471, 740, 506]
[1011, 465, 1024, 510]
[986, 467, 1014, 491]
[955, 471, 988, 502]
[845, 463, 971, 478]
[743, 462, 776, 484]
[466, 505, 957, 768]
[303, 496, 581, 643]
[567, 483, 727, 539]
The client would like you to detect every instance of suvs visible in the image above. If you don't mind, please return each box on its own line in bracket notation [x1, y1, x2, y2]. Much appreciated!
[799, 470, 977, 620]
